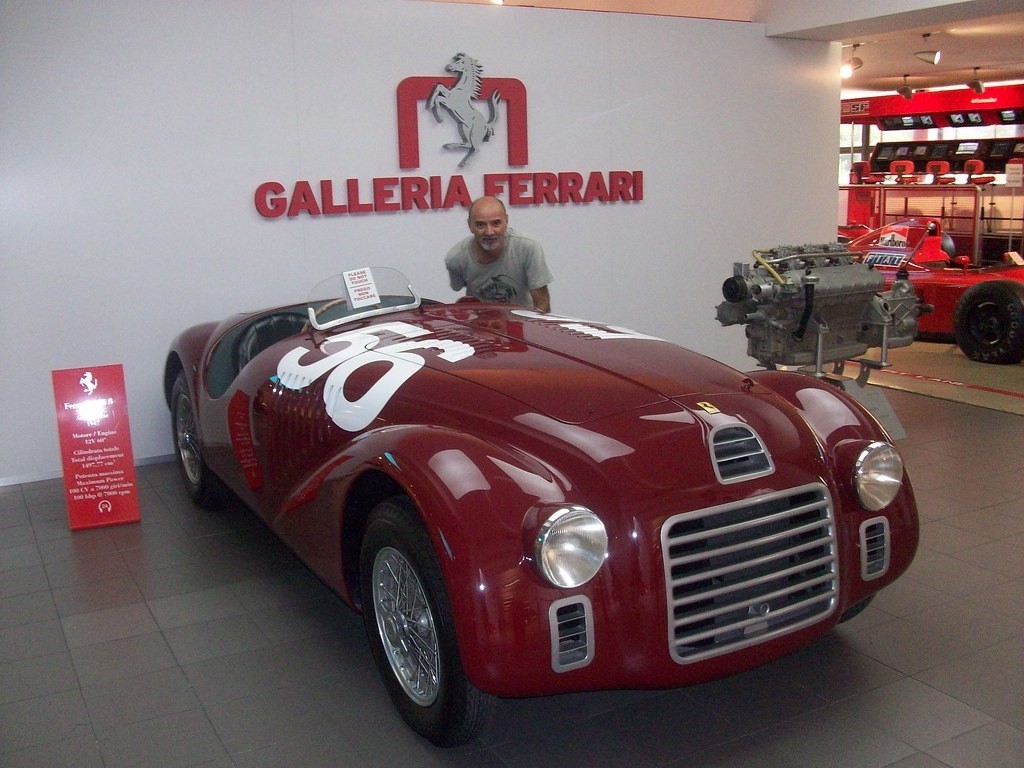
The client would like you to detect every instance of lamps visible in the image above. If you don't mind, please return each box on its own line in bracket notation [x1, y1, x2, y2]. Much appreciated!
[966, 68, 985, 94]
[841, 43, 862, 78]
[896, 75, 912, 99]
[915, 33, 940, 65]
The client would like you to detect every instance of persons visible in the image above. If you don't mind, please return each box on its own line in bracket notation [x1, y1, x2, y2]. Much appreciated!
[470, 309, 511, 340]
[445, 196, 554, 313]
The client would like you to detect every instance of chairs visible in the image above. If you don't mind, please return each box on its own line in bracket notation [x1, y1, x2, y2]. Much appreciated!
[851, 157, 1024, 185]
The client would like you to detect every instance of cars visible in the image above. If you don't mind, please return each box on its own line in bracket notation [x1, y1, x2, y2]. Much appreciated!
[164, 269, 920, 746]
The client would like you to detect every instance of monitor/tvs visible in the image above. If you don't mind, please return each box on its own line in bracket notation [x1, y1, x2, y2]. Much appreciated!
[878, 142, 1024, 160]
[879, 110, 1019, 129]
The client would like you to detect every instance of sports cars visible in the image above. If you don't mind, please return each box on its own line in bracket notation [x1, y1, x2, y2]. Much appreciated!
[838, 218, 1023, 363]
